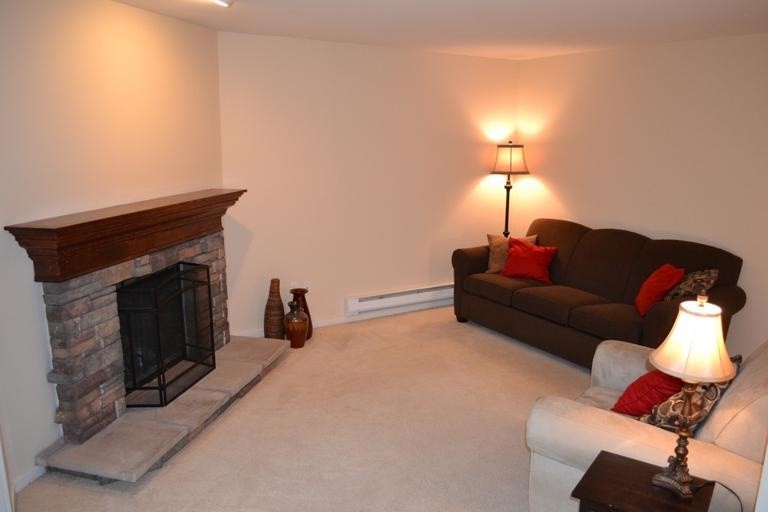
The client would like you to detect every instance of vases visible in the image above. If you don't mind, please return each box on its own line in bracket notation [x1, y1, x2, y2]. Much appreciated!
[263, 278, 313, 348]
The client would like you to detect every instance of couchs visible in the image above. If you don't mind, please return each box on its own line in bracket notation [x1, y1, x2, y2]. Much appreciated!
[525, 339, 767, 511]
[452, 218, 747, 369]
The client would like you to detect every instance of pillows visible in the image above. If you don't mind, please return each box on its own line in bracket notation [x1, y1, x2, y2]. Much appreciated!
[634, 263, 719, 317]
[610, 354, 742, 433]
[485, 233, 560, 285]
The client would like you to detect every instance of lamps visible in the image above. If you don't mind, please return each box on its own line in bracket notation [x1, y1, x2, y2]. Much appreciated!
[648, 295, 737, 474]
[489, 140, 530, 237]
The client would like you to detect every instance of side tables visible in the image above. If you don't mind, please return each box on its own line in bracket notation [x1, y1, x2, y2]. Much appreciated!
[571, 450, 715, 512]
